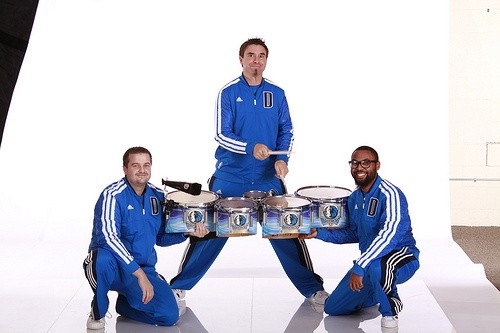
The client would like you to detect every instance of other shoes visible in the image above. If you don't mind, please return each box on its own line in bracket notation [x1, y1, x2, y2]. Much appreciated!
[176, 300, 186, 315]
[172, 289, 186, 300]
[87, 300, 112, 330]
[305, 289, 331, 304]
[381, 315, 398, 328]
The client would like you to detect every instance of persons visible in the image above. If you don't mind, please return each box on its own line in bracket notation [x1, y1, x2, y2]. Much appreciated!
[169, 37, 331, 306]
[81, 146, 209, 331]
[297, 146, 420, 328]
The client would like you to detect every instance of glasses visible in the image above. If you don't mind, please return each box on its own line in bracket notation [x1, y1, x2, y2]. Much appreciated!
[349, 159, 376, 169]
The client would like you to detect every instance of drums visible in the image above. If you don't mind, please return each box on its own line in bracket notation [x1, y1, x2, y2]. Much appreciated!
[164, 191, 222, 235]
[214, 196, 260, 238]
[296, 186, 352, 230]
[260, 196, 313, 238]
[244, 191, 267, 200]
